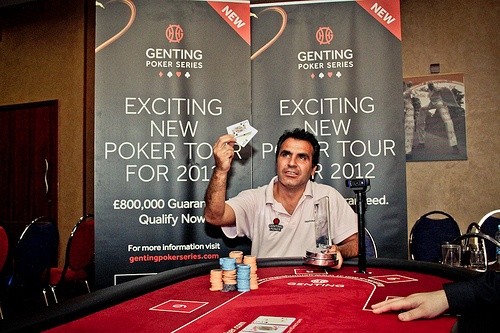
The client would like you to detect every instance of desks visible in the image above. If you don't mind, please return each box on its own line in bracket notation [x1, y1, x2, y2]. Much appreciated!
[1, 256, 481, 333]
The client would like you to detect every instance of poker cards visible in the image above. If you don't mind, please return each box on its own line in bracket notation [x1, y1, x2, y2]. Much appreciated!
[242, 324, 288, 333]
[368, 274, 418, 284]
[149, 300, 209, 313]
[226, 120, 258, 147]
[294, 268, 328, 275]
[252, 316, 296, 326]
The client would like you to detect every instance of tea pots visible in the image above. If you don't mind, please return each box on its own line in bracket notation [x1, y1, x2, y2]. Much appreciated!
[462, 223, 484, 269]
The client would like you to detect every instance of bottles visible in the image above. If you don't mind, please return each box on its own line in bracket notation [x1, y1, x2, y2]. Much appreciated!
[495, 225, 500, 271]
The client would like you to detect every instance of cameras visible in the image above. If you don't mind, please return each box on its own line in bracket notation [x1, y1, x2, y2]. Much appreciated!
[345, 178, 370, 188]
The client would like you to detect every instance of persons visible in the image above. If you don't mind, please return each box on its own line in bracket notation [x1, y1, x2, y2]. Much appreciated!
[204, 128, 359, 270]
[371, 272, 500, 321]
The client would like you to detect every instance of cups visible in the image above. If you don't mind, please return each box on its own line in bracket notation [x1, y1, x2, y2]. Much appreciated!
[442, 245, 461, 267]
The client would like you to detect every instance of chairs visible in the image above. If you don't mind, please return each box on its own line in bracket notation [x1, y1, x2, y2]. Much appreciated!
[16, 216, 57, 306]
[52, 216, 96, 293]
[475, 208, 500, 268]
[409, 211, 462, 263]
